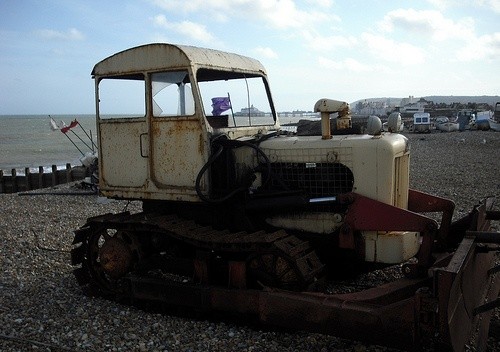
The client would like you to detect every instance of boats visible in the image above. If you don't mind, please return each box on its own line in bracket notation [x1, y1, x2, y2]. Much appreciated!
[241, 104, 264, 117]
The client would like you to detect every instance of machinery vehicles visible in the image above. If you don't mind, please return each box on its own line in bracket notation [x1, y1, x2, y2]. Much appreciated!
[68, 41, 500, 351]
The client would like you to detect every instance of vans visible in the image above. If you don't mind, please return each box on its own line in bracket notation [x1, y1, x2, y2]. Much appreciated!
[412, 112, 431, 133]
